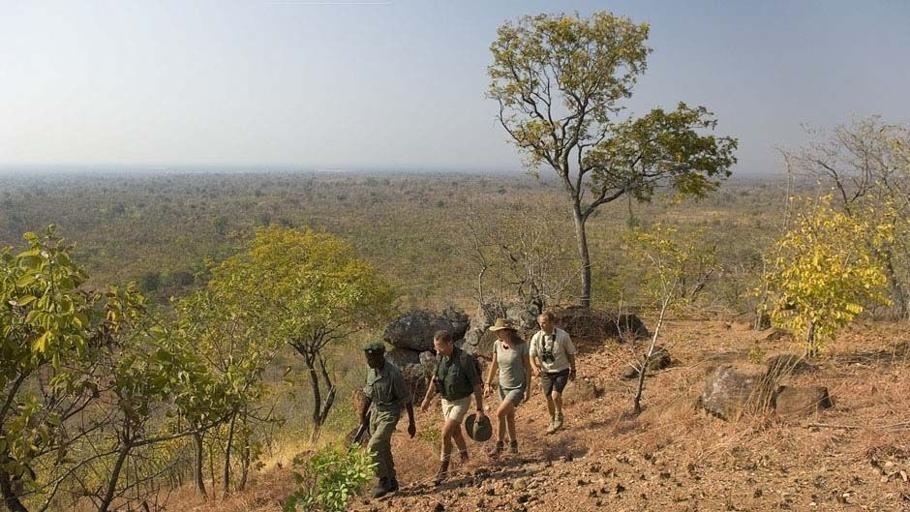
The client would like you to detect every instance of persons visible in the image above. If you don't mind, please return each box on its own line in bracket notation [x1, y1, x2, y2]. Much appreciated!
[530, 311, 578, 436]
[420, 330, 487, 486]
[363, 342, 416, 497]
[484, 317, 531, 458]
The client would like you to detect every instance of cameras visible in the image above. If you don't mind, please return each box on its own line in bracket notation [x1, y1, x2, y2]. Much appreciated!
[541, 353, 555, 363]
[433, 378, 445, 393]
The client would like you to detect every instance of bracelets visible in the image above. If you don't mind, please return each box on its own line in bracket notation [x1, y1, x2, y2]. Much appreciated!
[485, 381, 492, 386]
[407, 422, 415, 426]
[571, 369, 576, 372]
[476, 407, 483, 411]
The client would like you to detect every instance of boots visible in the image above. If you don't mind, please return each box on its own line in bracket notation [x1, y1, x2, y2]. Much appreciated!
[554, 412, 563, 429]
[546, 418, 555, 433]
[459, 450, 468, 462]
[488, 441, 504, 456]
[432, 460, 449, 482]
[510, 440, 518, 453]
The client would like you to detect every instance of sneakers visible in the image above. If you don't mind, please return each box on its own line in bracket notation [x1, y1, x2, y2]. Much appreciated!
[372, 476, 398, 498]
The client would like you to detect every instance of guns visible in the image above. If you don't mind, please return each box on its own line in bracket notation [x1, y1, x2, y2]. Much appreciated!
[347, 411, 371, 456]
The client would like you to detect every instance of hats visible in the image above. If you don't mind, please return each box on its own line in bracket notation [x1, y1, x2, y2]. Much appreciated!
[465, 414, 492, 441]
[488, 317, 518, 331]
[365, 341, 386, 354]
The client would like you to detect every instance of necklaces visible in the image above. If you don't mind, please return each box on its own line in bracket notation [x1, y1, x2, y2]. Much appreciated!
[503, 340, 515, 349]
